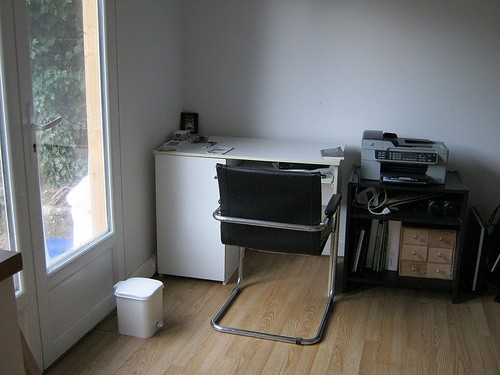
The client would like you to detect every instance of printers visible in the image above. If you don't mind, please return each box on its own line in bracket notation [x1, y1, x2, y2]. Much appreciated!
[360, 130, 448, 185]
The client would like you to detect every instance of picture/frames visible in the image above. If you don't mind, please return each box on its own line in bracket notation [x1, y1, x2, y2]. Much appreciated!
[181, 112, 198, 135]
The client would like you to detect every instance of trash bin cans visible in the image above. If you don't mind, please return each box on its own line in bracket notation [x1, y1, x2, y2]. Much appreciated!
[112, 277, 164, 339]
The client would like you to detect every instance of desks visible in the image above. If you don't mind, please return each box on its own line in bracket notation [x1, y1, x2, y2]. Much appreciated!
[1, 246, 40, 375]
[153, 135, 347, 285]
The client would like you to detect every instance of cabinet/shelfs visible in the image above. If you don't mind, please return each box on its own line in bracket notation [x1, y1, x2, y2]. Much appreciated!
[342, 165, 471, 304]
[398, 227, 457, 280]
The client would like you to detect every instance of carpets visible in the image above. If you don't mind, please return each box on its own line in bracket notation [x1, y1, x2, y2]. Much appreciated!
[44, 236, 76, 262]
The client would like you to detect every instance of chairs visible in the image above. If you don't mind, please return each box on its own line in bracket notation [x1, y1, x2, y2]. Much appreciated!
[209, 162, 342, 347]
